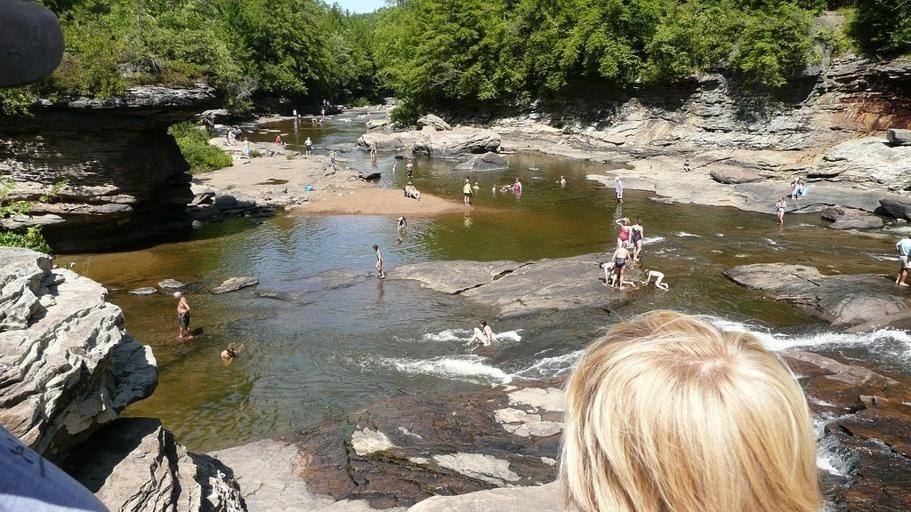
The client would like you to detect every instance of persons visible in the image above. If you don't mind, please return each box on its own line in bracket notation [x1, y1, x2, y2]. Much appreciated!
[218, 347, 235, 358]
[895, 232, 911, 286]
[598, 217, 668, 292]
[464, 210, 473, 233]
[372, 244, 384, 279]
[462, 176, 523, 205]
[558, 308, 825, 512]
[404, 180, 420, 199]
[396, 216, 408, 230]
[615, 176, 624, 203]
[559, 176, 566, 184]
[172, 292, 191, 338]
[201, 98, 413, 178]
[789, 176, 808, 199]
[775, 196, 785, 224]
